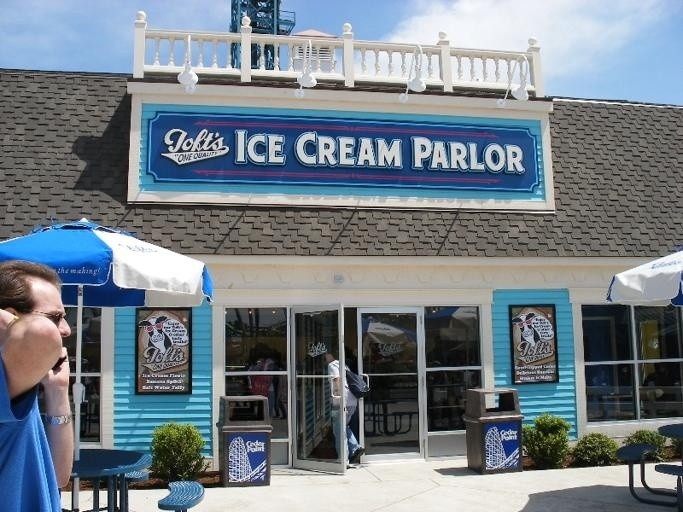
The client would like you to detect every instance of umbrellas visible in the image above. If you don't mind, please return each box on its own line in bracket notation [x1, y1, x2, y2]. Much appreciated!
[607, 250, 683, 307]
[363, 316, 416, 374]
[425, 307, 479, 365]
[0, 218, 213, 512]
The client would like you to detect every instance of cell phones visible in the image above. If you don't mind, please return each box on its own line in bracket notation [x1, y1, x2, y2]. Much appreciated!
[52, 358, 68, 372]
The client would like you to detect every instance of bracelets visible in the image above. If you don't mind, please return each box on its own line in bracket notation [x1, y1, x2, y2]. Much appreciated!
[45, 412, 72, 425]
[7, 315, 20, 330]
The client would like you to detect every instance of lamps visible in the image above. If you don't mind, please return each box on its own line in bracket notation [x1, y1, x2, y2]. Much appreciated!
[294, 39, 317, 98]
[176, 33, 199, 95]
[398, 44, 426, 103]
[497, 54, 530, 109]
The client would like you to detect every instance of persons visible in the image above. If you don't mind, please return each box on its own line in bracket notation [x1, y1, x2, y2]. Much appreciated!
[247, 354, 288, 420]
[322, 352, 366, 465]
[0, 260, 74, 512]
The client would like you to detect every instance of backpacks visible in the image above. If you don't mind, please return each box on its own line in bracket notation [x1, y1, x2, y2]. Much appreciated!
[346, 370, 371, 400]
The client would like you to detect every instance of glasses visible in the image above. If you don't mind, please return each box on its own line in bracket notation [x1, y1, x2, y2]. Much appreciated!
[18, 306, 70, 328]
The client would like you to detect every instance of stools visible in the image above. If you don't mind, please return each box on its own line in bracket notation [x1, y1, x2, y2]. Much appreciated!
[101, 471, 148, 511]
[159, 481, 205, 511]
[616, 442, 683, 511]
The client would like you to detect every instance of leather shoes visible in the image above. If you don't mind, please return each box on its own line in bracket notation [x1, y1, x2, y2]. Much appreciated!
[349, 447, 367, 464]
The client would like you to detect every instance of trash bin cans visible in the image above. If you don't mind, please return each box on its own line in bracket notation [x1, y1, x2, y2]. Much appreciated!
[462, 387, 524, 475]
[216, 395, 274, 487]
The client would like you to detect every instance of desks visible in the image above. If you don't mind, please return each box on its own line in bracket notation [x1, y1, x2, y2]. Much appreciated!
[658, 422, 683, 438]
[71, 447, 153, 512]
